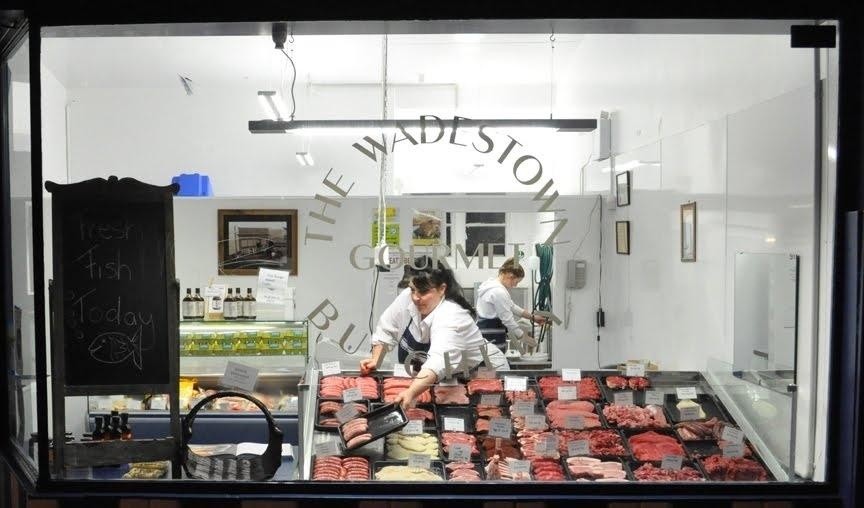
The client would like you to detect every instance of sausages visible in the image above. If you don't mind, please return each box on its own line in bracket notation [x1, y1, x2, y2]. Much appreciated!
[674, 417, 736, 440]
[383, 376, 432, 404]
[529, 461, 567, 482]
[312, 456, 369, 481]
[319, 404, 368, 425]
[342, 418, 371, 446]
[321, 376, 377, 400]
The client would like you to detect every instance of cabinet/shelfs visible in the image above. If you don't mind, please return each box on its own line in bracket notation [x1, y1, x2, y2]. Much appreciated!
[86, 317, 310, 446]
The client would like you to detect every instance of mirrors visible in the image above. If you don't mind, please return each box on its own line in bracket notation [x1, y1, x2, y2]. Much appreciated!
[732, 251, 796, 396]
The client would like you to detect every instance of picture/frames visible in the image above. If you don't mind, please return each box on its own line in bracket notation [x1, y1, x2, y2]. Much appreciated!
[216, 208, 298, 274]
[616, 221, 629, 254]
[680, 202, 696, 261]
[616, 171, 629, 206]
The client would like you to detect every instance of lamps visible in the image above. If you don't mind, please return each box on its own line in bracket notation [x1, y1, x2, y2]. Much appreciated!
[257, 57, 316, 168]
[245, 40, 600, 133]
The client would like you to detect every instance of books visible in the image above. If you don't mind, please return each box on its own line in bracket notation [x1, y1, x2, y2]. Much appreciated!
[186, 442, 295, 462]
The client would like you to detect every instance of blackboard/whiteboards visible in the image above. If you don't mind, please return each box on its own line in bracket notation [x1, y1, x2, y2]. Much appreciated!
[45, 174, 180, 396]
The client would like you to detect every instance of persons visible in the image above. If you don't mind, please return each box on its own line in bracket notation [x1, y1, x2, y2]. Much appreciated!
[360, 255, 510, 414]
[475, 257, 546, 355]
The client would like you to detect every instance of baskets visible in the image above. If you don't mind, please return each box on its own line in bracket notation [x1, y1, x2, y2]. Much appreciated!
[181, 391, 282, 481]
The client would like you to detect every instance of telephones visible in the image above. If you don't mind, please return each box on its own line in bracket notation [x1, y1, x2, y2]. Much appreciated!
[566, 260, 587, 289]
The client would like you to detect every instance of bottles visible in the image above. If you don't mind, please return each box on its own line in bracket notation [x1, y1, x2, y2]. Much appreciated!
[92, 410, 133, 468]
[235, 288, 243, 318]
[223, 288, 237, 319]
[242, 288, 257, 319]
[28, 432, 92, 468]
[193, 288, 205, 319]
[182, 288, 196, 318]
